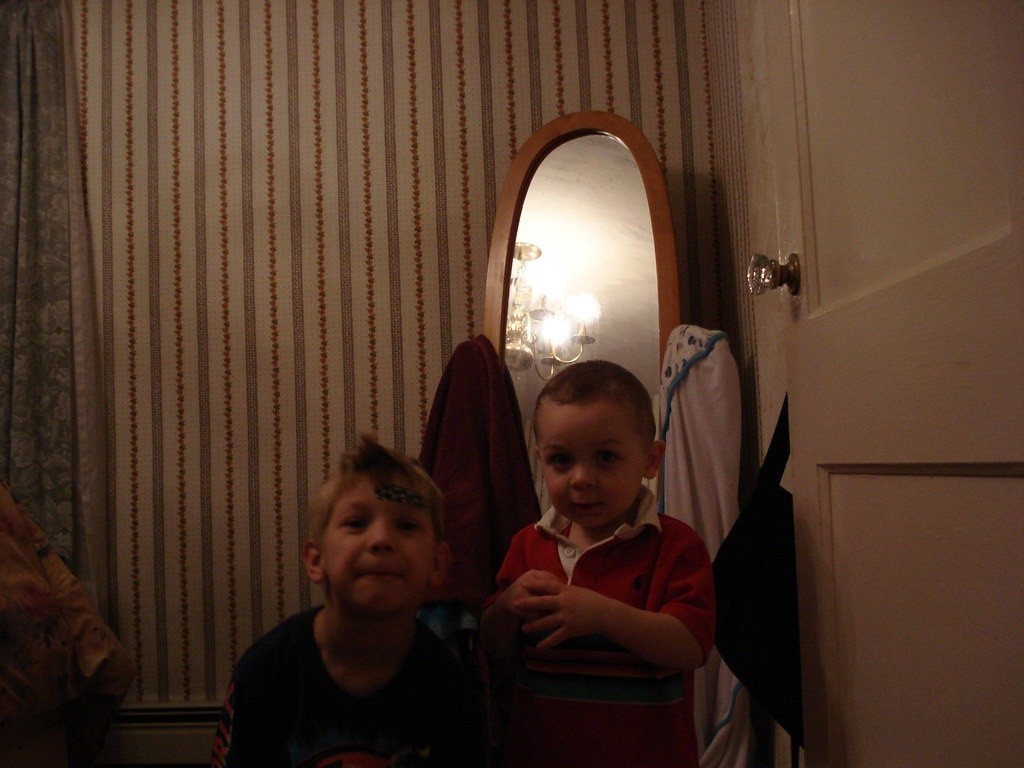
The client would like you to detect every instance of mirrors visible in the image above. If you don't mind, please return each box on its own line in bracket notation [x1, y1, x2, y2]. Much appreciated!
[484, 110, 692, 521]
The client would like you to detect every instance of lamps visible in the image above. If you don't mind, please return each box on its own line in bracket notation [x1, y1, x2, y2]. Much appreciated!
[505, 242, 595, 380]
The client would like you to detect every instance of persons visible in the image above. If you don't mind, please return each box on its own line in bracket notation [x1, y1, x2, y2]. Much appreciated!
[0, 474, 133, 768]
[479, 360, 717, 768]
[208, 432, 490, 768]
